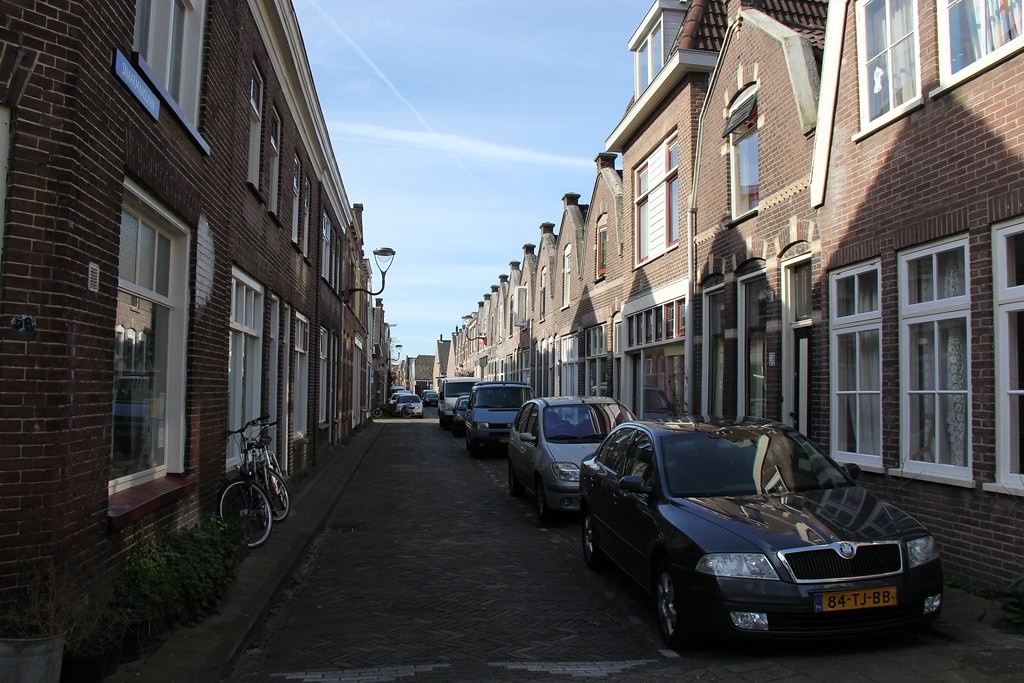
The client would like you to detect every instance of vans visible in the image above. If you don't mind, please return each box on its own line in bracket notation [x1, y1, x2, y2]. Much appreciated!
[437, 377, 482, 429]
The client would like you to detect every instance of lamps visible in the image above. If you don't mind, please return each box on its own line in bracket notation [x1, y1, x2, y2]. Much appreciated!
[460, 314, 487, 345]
[386, 344, 403, 363]
[344, 247, 396, 304]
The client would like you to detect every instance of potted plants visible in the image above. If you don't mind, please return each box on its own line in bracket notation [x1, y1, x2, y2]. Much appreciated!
[0, 436, 154, 683]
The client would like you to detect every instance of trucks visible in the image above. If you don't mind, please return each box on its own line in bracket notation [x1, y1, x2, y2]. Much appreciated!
[115, 374, 161, 454]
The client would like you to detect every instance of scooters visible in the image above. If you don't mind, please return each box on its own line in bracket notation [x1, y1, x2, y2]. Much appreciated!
[371, 398, 414, 420]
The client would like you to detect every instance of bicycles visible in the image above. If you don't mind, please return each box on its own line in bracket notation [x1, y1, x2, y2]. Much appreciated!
[216, 413, 291, 548]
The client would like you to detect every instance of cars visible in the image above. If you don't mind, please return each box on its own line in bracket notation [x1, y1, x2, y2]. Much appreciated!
[578, 411, 945, 654]
[505, 395, 646, 524]
[451, 394, 471, 438]
[388, 386, 438, 419]
[588, 382, 678, 422]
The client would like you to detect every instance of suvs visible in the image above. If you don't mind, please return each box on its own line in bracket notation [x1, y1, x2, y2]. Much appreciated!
[464, 380, 537, 459]
